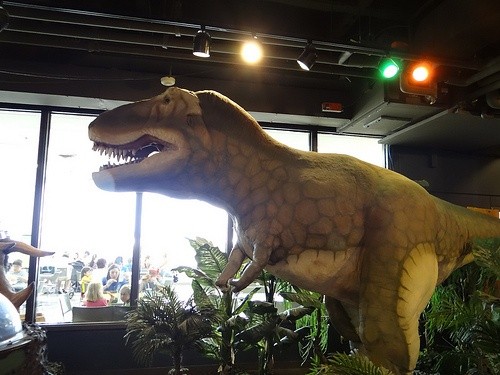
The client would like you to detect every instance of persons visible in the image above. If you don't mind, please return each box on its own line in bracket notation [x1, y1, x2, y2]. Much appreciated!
[81, 256, 131, 306]
[5, 260, 28, 292]
[56, 251, 92, 294]
[139, 255, 164, 291]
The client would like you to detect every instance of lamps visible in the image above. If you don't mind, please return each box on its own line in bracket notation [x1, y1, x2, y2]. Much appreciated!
[377, 55, 433, 82]
[193, 31, 212, 58]
[297, 46, 317, 71]
